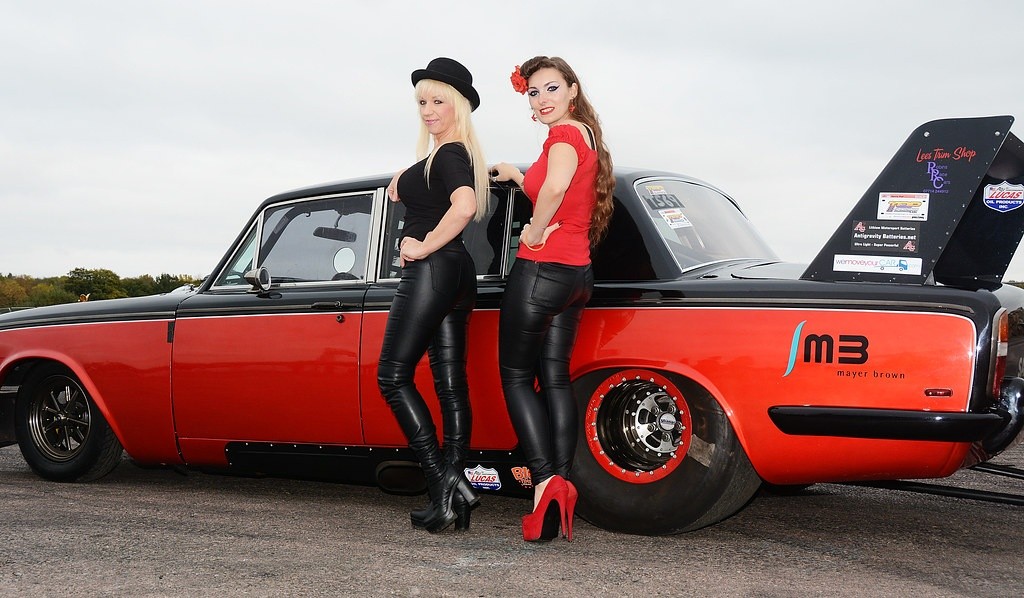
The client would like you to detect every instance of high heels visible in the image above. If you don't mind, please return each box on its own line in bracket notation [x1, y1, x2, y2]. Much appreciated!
[564, 481, 578, 544]
[424, 463, 480, 534]
[522, 476, 567, 542]
[409, 487, 474, 532]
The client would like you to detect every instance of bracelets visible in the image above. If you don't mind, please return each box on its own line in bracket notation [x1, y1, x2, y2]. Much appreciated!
[525, 233, 546, 251]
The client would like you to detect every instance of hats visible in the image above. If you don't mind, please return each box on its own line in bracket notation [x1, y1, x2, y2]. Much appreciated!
[411, 57, 480, 112]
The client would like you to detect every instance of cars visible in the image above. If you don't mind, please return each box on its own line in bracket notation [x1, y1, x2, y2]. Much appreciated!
[0, 113, 1023, 537]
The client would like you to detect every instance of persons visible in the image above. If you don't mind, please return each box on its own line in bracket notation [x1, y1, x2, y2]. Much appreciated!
[375, 58, 491, 533]
[486, 56, 617, 541]
